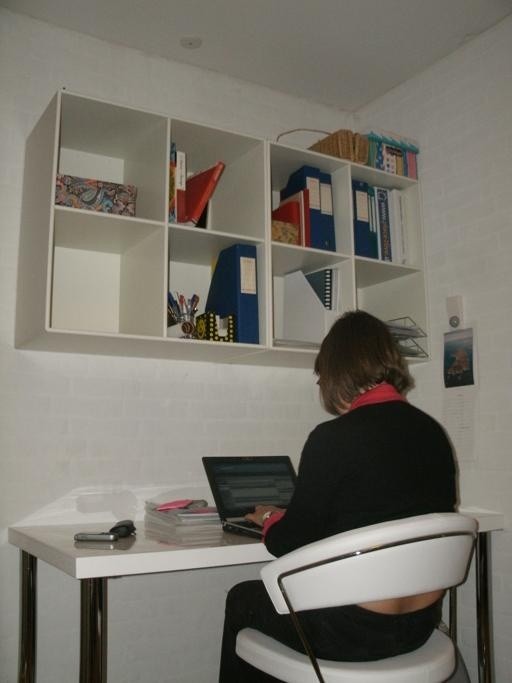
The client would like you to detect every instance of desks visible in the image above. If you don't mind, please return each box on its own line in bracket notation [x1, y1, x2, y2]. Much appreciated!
[8, 511, 507, 683]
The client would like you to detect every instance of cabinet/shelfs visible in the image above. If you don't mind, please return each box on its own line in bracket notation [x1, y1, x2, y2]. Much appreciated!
[14, 91, 433, 369]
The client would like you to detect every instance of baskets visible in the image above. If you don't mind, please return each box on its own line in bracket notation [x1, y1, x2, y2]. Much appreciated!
[306, 126, 369, 164]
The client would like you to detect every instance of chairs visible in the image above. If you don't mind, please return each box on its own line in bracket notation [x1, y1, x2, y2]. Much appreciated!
[234, 514, 480, 683]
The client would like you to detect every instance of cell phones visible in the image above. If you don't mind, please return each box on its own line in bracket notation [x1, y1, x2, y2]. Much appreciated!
[74, 531, 120, 542]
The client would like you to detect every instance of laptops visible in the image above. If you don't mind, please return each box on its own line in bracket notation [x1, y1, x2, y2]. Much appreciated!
[201, 455, 298, 540]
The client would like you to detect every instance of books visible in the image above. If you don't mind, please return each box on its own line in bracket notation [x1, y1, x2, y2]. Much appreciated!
[184, 160, 226, 227]
[168, 141, 175, 224]
[384, 323, 421, 338]
[268, 128, 422, 267]
[143, 497, 222, 529]
[145, 525, 225, 547]
[304, 267, 333, 311]
[329, 268, 340, 310]
[194, 198, 212, 229]
[176, 149, 186, 222]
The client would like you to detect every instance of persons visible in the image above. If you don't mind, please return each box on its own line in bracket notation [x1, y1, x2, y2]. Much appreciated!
[211, 308, 464, 683]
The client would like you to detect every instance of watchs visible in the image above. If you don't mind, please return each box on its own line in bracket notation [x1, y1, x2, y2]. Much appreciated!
[261, 510, 275, 524]
